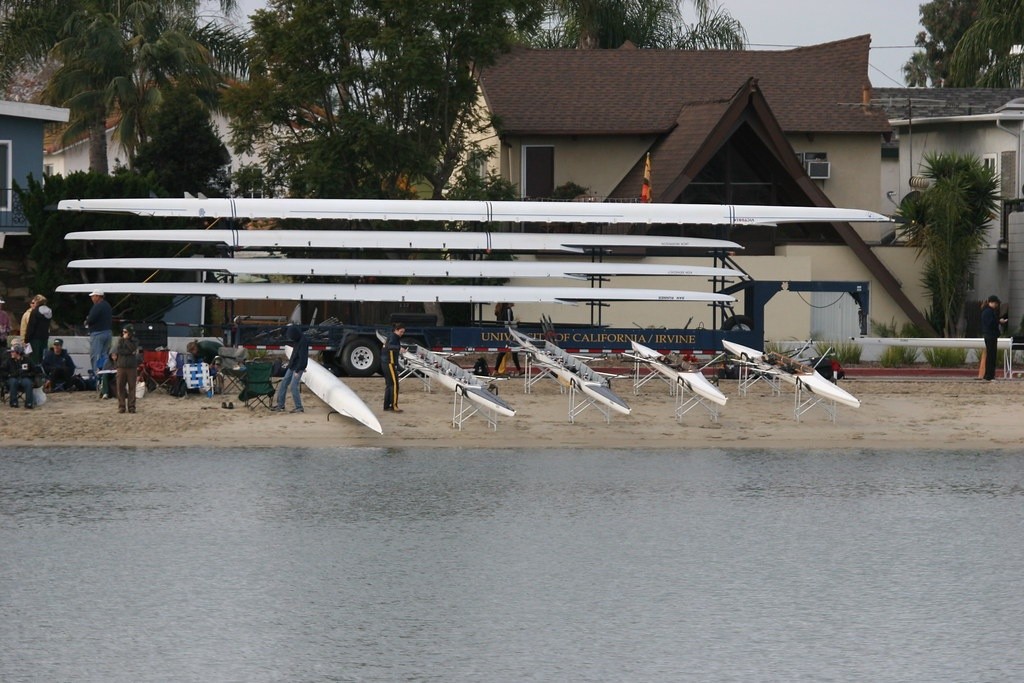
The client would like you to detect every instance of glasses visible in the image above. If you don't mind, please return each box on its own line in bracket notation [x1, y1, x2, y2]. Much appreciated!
[31, 302, 34, 304]
[123, 331, 128, 333]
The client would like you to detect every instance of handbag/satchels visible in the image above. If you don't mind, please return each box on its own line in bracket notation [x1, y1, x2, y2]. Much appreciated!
[126, 380, 146, 399]
[23, 386, 48, 407]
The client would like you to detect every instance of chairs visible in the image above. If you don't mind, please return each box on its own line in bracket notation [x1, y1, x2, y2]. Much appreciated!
[40, 349, 67, 392]
[211, 354, 281, 411]
[0, 381, 36, 406]
[141, 350, 183, 399]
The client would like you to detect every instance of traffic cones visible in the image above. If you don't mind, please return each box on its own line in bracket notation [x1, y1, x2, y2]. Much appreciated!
[974, 349, 987, 380]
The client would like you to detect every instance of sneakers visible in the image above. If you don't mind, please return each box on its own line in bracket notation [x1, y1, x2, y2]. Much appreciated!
[102, 394, 108, 399]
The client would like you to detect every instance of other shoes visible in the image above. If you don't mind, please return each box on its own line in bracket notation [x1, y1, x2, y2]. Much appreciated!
[289, 407, 304, 413]
[270, 406, 285, 412]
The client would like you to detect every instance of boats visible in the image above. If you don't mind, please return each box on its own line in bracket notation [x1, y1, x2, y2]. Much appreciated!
[56, 283, 737, 307]
[721, 338, 861, 409]
[376, 328, 517, 417]
[68, 260, 747, 277]
[284, 345, 385, 435]
[64, 231, 746, 254]
[631, 340, 729, 406]
[508, 326, 632, 415]
[58, 198, 896, 227]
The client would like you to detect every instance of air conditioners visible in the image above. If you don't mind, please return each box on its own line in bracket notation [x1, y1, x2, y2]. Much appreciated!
[806, 161, 831, 179]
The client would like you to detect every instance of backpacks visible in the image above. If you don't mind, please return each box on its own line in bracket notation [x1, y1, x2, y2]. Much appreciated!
[170, 379, 188, 397]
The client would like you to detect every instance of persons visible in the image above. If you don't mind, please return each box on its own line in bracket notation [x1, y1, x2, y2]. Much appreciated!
[116, 323, 139, 414]
[981, 295, 1008, 383]
[270, 326, 309, 413]
[0, 294, 53, 409]
[83, 290, 114, 389]
[186, 340, 223, 380]
[380, 322, 405, 413]
[102, 346, 117, 399]
[40, 338, 76, 393]
[491, 303, 525, 376]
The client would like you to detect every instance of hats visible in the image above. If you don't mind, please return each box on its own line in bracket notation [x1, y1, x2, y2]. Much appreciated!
[54, 339, 63, 346]
[6, 345, 22, 352]
[89, 288, 104, 296]
[11, 339, 21, 345]
